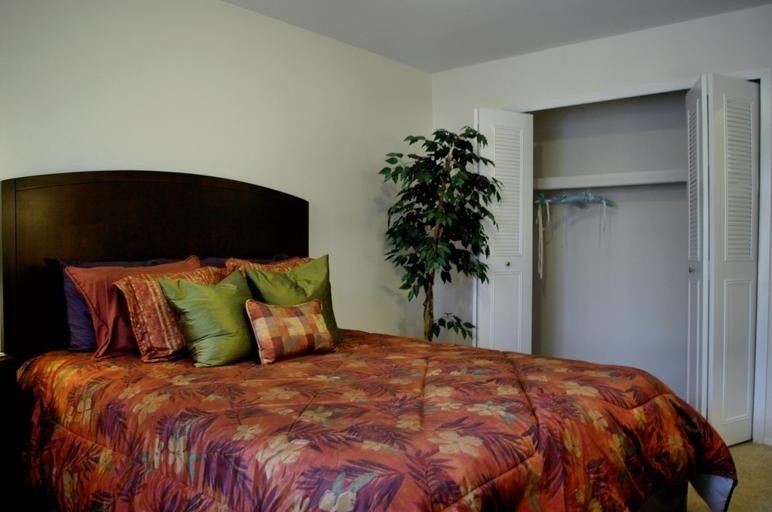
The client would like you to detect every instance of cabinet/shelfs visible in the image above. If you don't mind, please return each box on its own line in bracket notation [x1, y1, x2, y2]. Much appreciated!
[469, 76, 760, 450]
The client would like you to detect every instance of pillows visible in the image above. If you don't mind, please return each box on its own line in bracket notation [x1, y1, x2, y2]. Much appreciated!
[60, 255, 343, 368]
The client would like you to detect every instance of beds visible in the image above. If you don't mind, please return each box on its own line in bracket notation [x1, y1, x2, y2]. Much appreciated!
[0, 171, 736, 512]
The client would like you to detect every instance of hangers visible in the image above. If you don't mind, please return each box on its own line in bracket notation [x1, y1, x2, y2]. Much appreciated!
[534, 189, 613, 210]
